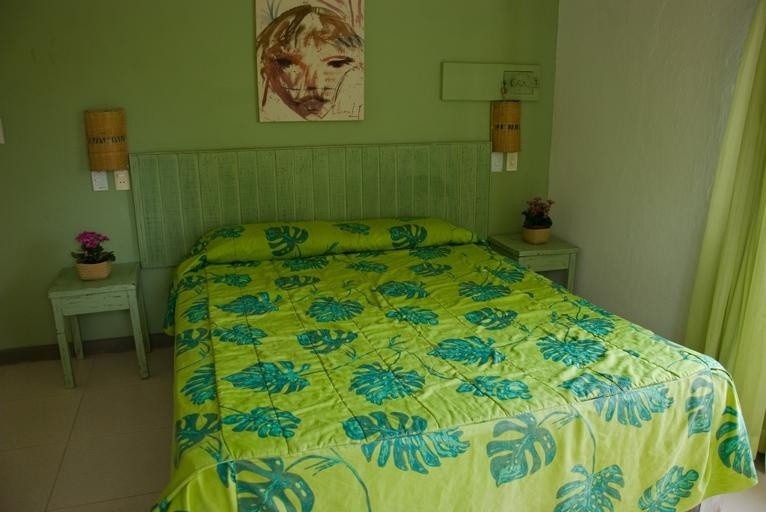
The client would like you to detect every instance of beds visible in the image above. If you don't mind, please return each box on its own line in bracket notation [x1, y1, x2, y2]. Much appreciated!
[129, 141, 758, 512]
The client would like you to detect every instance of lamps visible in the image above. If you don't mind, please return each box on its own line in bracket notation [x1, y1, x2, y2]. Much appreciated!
[491, 102, 521, 153]
[85, 107, 130, 172]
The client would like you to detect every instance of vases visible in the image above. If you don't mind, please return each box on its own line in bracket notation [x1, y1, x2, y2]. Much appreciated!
[75, 262, 112, 280]
[523, 228, 551, 244]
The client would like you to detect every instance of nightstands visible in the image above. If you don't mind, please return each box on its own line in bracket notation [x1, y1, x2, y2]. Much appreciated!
[47, 262, 151, 389]
[489, 234, 578, 292]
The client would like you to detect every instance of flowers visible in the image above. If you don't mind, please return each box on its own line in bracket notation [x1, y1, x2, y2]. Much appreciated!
[70, 230, 116, 263]
[520, 198, 555, 227]
